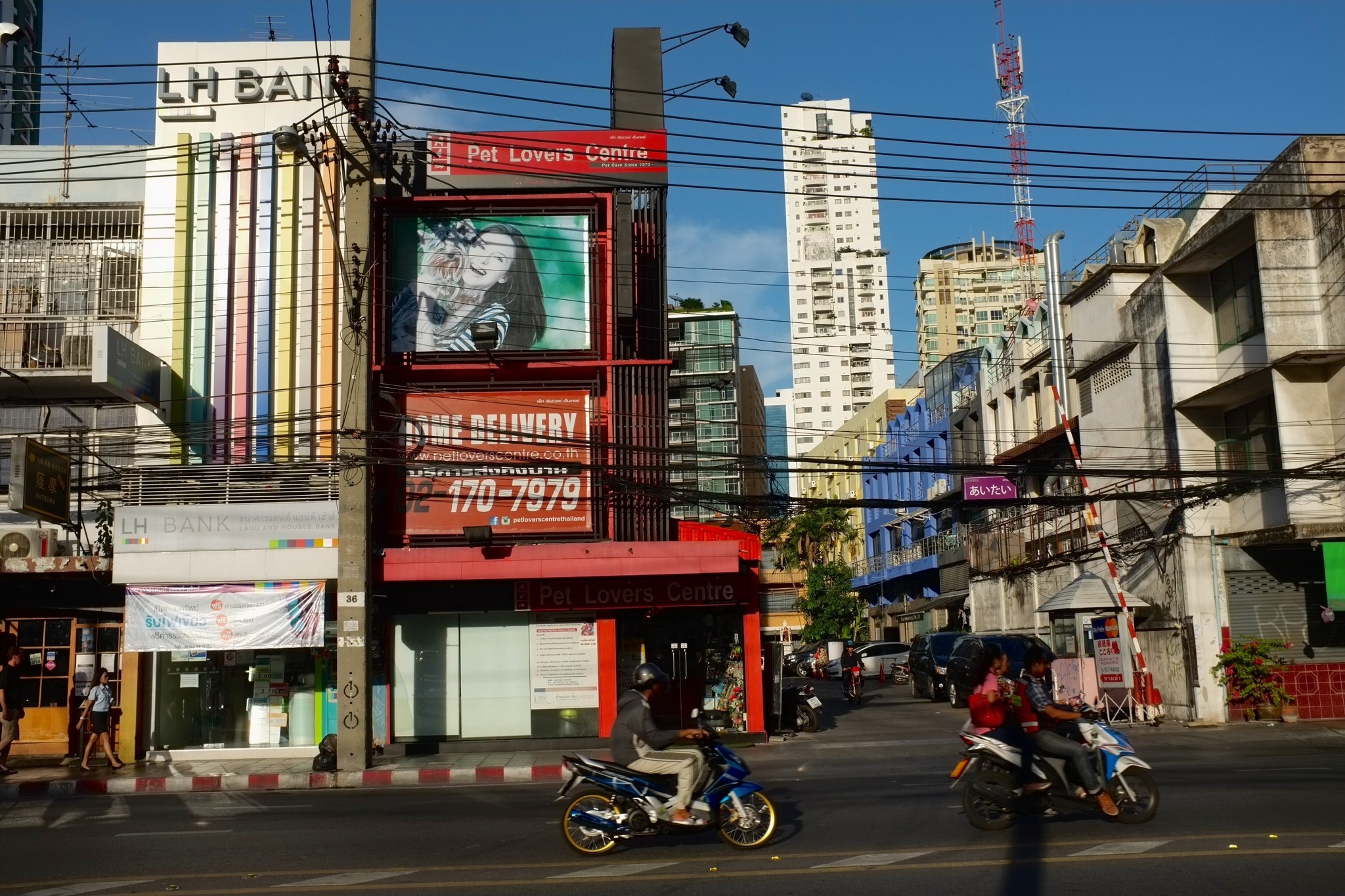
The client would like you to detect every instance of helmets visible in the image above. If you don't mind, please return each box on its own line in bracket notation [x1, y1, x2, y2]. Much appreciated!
[846, 640, 855, 649]
[633, 663, 665, 689]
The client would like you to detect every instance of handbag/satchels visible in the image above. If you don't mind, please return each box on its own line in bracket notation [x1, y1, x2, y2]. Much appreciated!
[814, 652, 818, 658]
[968, 676, 1007, 727]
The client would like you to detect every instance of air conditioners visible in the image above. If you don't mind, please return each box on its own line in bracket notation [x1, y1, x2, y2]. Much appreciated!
[845, 492, 851, 500]
[927, 486, 936, 500]
[60, 334, 93, 368]
[890, 554, 900, 567]
[808, 480, 816, 490]
[827, 468, 834, 477]
[825, 473, 830, 478]
[849, 324, 871, 410]
[801, 488, 806, 495]
[848, 491, 855, 499]
[827, 271, 837, 330]
[901, 550, 912, 563]
[0, 528, 58, 558]
[934, 479, 946, 496]
[844, 456, 854, 467]
[866, 442, 874, 452]
[894, 499, 905, 514]
[799, 144, 830, 231]
[912, 546, 922, 561]
[810, 268, 818, 332]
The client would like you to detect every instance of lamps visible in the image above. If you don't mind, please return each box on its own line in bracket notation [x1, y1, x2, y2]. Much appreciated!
[461, 524, 494, 548]
[661, 21, 749, 55]
[663, 75, 738, 104]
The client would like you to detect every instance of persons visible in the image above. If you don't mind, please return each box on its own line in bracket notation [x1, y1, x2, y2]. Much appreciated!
[611, 662, 710, 826]
[0, 646, 25, 774]
[392, 216, 547, 350]
[970, 643, 1119, 816]
[841, 644, 867, 693]
[75, 667, 126, 771]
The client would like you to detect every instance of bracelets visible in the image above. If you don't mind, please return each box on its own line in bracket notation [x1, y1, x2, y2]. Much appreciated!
[80, 717, 85, 720]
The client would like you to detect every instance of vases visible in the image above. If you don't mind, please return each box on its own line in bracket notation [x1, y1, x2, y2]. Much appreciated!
[1255, 703, 1284, 722]
[1281, 706, 1299, 723]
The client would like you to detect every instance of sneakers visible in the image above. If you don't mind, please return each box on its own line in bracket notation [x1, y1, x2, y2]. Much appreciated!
[1094, 789, 1119, 815]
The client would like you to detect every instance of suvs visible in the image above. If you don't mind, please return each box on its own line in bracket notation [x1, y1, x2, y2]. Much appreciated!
[908, 631, 977, 703]
[781, 637, 912, 681]
[945, 633, 1057, 708]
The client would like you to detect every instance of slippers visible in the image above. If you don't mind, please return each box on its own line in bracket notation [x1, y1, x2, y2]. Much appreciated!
[672, 815, 708, 826]
[1, 768, 18, 775]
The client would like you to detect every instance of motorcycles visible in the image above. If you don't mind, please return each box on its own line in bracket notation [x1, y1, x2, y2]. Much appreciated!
[782, 683, 825, 732]
[889, 661, 911, 685]
[554, 709, 775, 856]
[841, 666, 865, 705]
[947, 695, 1160, 832]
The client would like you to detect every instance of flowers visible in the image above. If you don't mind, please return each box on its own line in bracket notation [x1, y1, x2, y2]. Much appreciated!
[1210, 634, 1297, 718]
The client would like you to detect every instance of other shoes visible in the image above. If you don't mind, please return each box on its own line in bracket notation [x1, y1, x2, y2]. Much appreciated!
[1025, 782, 1052, 795]
[844, 690, 848, 696]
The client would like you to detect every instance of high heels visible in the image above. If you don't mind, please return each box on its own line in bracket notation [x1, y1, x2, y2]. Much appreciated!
[111, 764, 126, 771]
[80, 765, 94, 772]
